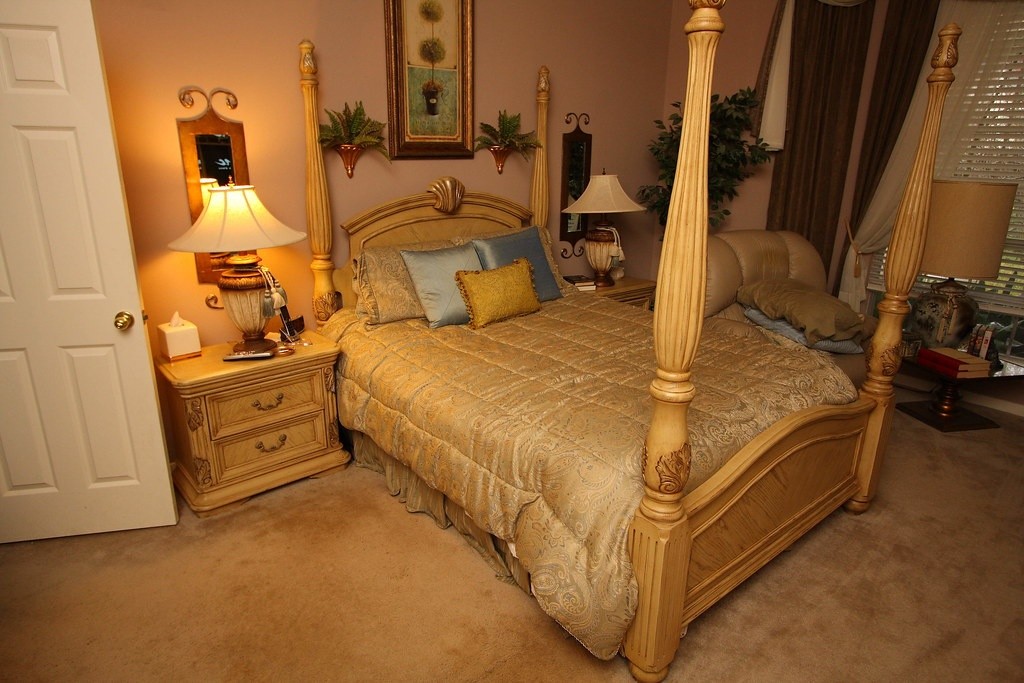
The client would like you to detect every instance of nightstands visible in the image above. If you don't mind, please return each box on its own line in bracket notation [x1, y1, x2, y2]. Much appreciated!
[583, 277, 657, 312]
[152, 328, 351, 518]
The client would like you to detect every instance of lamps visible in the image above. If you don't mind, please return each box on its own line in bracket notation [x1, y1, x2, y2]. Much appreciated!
[900, 179, 1019, 356]
[165, 176, 309, 353]
[561, 168, 647, 288]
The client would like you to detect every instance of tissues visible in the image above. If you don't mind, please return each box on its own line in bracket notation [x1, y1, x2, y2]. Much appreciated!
[156, 310, 203, 364]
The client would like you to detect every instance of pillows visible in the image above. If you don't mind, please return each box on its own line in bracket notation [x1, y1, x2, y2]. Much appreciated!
[349, 222, 575, 328]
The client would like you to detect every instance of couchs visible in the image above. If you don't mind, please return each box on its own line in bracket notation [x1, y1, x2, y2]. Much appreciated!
[701, 228, 880, 355]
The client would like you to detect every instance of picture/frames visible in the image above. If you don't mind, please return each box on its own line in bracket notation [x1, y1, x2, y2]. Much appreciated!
[383, 0, 475, 161]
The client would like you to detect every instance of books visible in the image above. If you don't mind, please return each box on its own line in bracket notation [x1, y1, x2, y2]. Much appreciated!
[563, 274, 596, 292]
[915, 324, 996, 378]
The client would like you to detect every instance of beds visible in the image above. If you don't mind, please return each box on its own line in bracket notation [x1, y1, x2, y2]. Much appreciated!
[297, 0, 963, 683]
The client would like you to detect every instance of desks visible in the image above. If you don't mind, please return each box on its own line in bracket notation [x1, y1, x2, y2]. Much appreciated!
[893, 345, 1024, 432]
